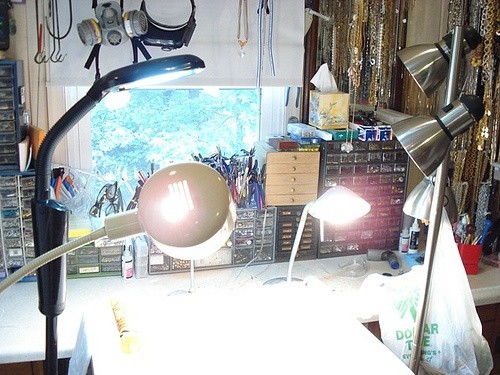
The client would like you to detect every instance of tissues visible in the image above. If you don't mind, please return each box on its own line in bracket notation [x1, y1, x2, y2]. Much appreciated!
[308, 62, 350, 130]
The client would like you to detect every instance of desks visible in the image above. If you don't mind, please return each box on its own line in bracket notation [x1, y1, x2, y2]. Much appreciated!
[83, 309, 416, 375]
[0, 240, 500, 375]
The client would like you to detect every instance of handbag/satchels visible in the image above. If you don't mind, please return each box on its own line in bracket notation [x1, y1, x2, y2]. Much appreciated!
[362, 206, 495, 374]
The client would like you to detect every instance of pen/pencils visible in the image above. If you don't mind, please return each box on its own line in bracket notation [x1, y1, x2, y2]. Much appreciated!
[457, 212, 491, 246]
[137, 163, 154, 187]
[190, 145, 266, 209]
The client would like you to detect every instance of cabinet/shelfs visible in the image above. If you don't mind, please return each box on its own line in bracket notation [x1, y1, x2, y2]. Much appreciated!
[0, 58, 125, 283]
[147, 131, 410, 275]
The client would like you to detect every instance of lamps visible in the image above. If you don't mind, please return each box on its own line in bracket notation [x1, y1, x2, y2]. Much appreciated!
[391, 94, 485, 178]
[396, 25, 480, 98]
[398, 156, 460, 225]
[30, 54, 207, 374]
[262, 185, 370, 285]
[0, 162, 237, 293]
